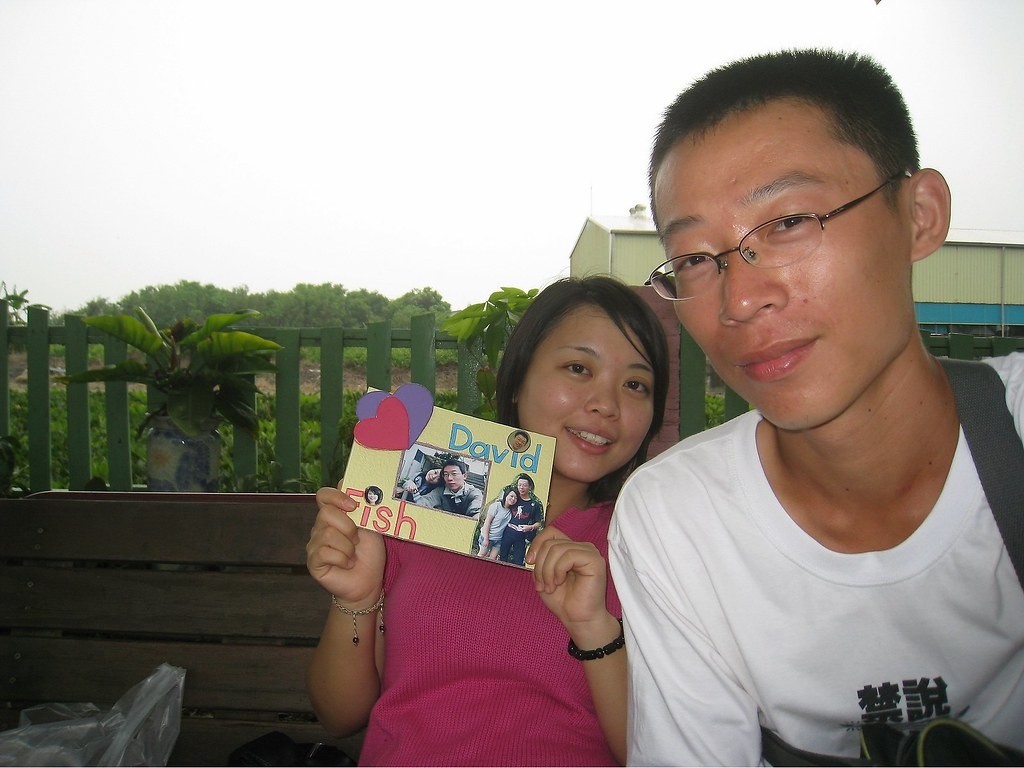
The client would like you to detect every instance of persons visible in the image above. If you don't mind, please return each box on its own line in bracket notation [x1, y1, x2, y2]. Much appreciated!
[609, 50, 1024, 767]
[499, 477, 541, 565]
[415, 459, 482, 518]
[403, 466, 444, 502]
[478, 486, 519, 560]
[366, 487, 381, 505]
[305, 274, 669, 768]
[513, 432, 529, 450]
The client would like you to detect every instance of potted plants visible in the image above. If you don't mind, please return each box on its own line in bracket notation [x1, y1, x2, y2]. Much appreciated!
[50, 299, 288, 491]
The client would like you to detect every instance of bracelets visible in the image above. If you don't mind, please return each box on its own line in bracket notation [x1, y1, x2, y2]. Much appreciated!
[332, 589, 385, 646]
[567, 617, 624, 661]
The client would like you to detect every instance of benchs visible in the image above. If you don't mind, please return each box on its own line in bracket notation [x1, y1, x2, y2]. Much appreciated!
[0, 501, 366, 766]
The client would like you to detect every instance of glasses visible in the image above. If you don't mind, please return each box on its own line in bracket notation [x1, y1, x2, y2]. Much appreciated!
[644, 168, 913, 301]
